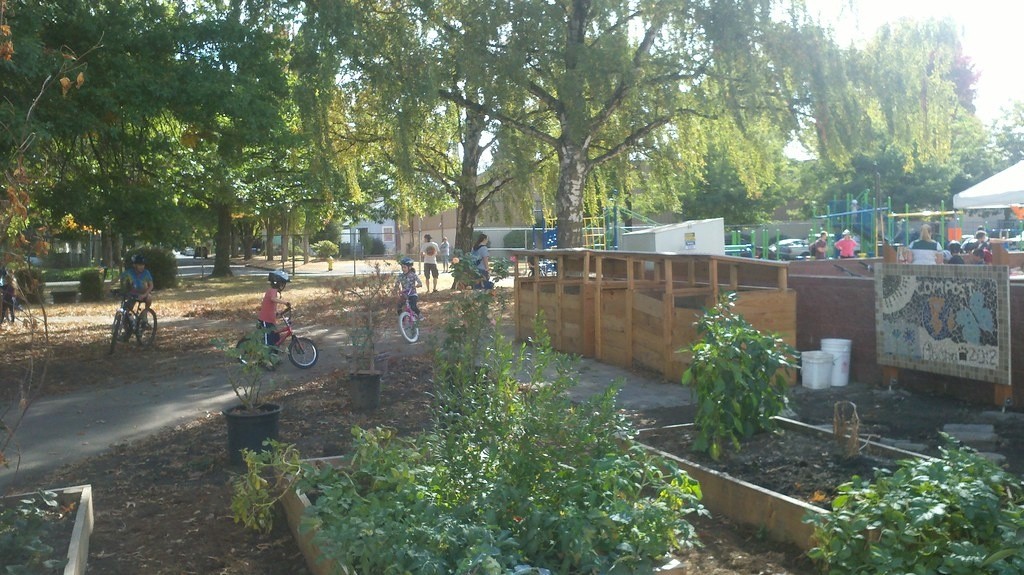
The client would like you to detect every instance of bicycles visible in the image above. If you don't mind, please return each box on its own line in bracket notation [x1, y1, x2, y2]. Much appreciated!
[393, 286, 420, 343]
[236, 304, 318, 369]
[527, 259, 557, 277]
[109, 289, 157, 355]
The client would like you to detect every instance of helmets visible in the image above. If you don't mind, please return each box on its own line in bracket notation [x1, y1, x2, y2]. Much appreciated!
[268, 270, 291, 290]
[399, 258, 414, 268]
[131, 253, 148, 264]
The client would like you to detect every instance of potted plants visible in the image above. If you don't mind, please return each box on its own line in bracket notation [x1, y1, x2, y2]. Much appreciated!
[330, 255, 416, 413]
[209, 324, 294, 469]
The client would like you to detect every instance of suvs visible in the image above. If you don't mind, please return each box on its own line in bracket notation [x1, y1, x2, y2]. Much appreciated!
[811, 234, 861, 257]
[194, 247, 208, 259]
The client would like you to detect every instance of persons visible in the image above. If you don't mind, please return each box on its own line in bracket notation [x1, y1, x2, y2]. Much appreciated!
[420, 234, 441, 294]
[439, 237, 450, 274]
[0, 266, 14, 323]
[392, 257, 424, 326]
[469, 234, 494, 290]
[99, 261, 108, 282]
[257, 271, 292, 371]
[834, 229, 856, 259]
[906, 224, 943, 266]
[104, 254, 154, 342]
[809, 231, 828, 259]
[528, 248, 540, 277]
[948, 225, 992, 264]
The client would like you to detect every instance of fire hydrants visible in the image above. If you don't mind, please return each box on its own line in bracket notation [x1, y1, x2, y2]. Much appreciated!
[327, 256, 334, 271]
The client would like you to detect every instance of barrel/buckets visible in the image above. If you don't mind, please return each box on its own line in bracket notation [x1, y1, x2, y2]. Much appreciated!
[801, 338, 852, 389]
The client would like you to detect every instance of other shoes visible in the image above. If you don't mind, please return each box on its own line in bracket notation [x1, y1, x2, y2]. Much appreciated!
[442, 271, 449, 273]
[418, 313, 424, 322]
[262, 360, 275, 371]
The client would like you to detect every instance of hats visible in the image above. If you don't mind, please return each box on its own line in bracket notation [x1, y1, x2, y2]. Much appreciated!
[842, 228, 851, 237]
[424, 233, 432, 239]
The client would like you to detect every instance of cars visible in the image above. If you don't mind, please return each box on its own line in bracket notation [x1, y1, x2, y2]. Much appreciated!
[181, 247, 194, 255]
[770, 239, 810, 257]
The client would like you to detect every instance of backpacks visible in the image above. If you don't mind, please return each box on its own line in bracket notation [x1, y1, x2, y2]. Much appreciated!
[808, 242, 816, 256]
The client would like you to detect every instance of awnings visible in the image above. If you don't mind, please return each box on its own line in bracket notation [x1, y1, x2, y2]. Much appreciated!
[952, 160, 1024, 209]
